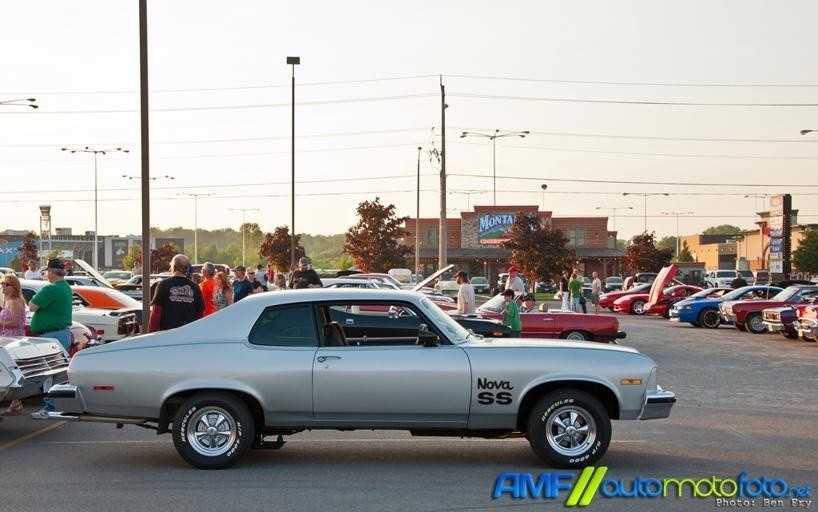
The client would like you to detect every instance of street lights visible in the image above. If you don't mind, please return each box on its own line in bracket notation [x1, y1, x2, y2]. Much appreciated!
[410, 145, 427, 285]
[743, 194, 768, 267]
[800, 128, 817, 135]
[284, 56, 301, 269]
[1, 93, 40, 111]
[62, 145, 265, 272]
[595, 189, 699, 261]
[458, 125, 532, 210]
[539, 183, 552, 208]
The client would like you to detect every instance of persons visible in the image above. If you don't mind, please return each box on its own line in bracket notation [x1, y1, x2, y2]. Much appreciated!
[730, 272, 747, 289]
[505, 265, 525, 294]
[1, 256, 74, 419]
[453, 271, 476, 314]
[520, 293, 539, 313]
[499, 289, 522, 338]
[764, 290, 772, 299]
[132, 254, 323, 335]
[751, 290, 762, 300]
[559, 269, 601, 313]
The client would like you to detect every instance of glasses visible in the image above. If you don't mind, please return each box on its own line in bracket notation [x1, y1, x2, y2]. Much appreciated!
[0, 282, 16, 288]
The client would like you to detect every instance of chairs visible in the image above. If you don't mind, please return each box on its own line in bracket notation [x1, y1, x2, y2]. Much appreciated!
[538, 303, 547, 312]
[325, 321, 344, 346]
[329, 321, 346, 346]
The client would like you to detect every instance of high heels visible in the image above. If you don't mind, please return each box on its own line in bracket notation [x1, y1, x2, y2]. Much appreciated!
[0, 402, 25, 417]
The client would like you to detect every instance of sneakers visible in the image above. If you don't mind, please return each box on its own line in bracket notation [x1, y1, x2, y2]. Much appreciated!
[30, 408, 51, 421]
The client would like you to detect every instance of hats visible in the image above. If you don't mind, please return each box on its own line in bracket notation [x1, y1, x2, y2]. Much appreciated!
[499, 288, 514, 296]
[506, 266, 519, 273]
[41, 259, 65, 273]
[230, 265, 245, 273]
[453, 271, 467, 278]
